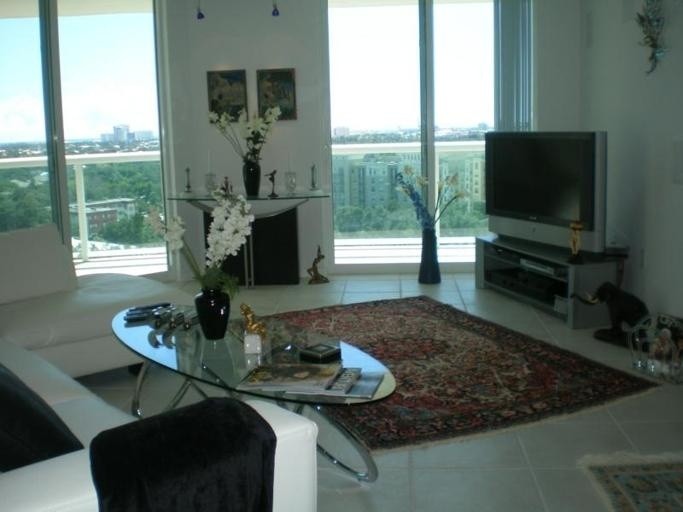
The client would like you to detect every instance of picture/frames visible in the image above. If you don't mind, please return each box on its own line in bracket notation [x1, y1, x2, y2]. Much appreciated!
[206, 67, 250, 125]
[257, 66, 299, 121]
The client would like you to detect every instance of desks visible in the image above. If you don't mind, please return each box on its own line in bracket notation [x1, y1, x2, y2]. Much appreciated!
[166, 187, 331, 289]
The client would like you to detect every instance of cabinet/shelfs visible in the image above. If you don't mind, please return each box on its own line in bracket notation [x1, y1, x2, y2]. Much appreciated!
[473, 235, 630, 329]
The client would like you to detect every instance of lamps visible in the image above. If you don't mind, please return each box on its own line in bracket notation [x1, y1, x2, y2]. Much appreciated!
[272, 3, 280, 17]
[196, 6, 204, 19]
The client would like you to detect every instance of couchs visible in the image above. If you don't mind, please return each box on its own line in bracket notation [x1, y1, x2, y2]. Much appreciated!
[0, 335, 317, 512]
[0, 219, 181, 379]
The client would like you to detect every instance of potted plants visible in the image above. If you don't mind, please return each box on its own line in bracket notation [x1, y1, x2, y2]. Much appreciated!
[397, 158, 471, 284]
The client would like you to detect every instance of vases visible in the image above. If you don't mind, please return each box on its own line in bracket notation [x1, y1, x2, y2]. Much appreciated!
[241, 158, 261, 196]
[193, 286, 231, 342]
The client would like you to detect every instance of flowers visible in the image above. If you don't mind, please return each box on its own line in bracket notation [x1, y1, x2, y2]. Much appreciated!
[151, 189, 256, 296]
[204, 105, 281, 161]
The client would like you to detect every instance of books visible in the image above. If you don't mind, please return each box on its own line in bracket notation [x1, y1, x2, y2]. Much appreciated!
[235, 362, 384, 399]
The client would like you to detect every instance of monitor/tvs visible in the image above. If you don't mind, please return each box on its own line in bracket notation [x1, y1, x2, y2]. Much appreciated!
[486, 131, 606, 252]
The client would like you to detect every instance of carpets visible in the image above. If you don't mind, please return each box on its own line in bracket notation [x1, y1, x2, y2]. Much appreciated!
[577, 448, 683, 512]
[222, 294, 664, 455]
